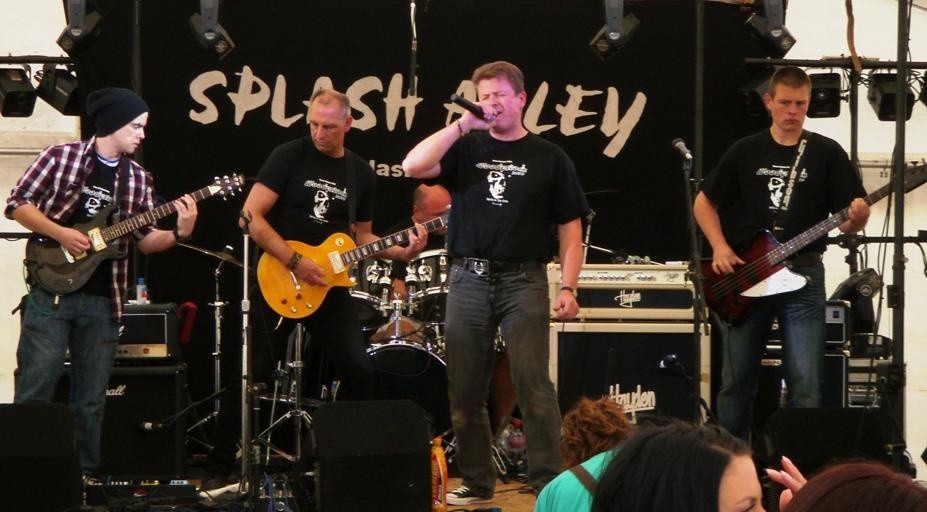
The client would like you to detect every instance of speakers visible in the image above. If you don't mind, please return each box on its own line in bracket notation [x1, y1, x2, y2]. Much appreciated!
[750, 407, 905, 512]
[1, 403, 87, 512]
[310, 400, 433, 512]
[63, 361, 189, 477]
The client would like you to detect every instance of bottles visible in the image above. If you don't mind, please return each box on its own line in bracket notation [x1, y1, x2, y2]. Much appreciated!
[137, 277, 147, 307]
[508, 419, 524, 479]
[432, 438, 449, 512]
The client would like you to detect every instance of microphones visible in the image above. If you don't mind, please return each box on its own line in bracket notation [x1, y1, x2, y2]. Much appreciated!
[669, 136, 692, 162]
[451, 93, 497, 124]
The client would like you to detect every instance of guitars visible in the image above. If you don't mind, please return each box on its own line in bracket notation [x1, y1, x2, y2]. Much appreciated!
[256, 215, 445, 321]
[20, 171, 246, 296]
[688, 180, 892, 326]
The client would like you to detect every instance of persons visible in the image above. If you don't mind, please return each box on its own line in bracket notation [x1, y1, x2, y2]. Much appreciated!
[3, 89, 198, 502]
[534, 394, 635, 512]
[360, 184, 452, 411]
[784, 461, 926, 511]
[242, 89, 428, 459]
[693, 67, 870, 475]
[589, 415, 809, 512]
[402, 62, 588, 506]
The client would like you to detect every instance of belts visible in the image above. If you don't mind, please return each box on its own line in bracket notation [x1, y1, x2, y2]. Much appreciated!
[451, 256, 548, 276]
[786, 251, 823, 267]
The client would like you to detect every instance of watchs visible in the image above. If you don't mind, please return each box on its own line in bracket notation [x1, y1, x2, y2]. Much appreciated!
[171, 228, 190, 244]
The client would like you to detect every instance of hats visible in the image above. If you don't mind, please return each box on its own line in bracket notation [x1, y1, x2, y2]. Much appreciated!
[86, 87, 153, 136]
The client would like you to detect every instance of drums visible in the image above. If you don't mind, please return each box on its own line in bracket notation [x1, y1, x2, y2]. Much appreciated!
[346, 253, 394, 315]
[405, 246, 449, 312]
[316, 318, 455, 448]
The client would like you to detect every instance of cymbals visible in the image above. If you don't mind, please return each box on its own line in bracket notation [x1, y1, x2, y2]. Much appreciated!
[582, 188, 623, 204]
[171, 239, 245, 273]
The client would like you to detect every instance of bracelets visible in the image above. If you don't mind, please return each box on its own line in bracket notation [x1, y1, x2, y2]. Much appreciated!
[286, 251, 302, 271]
[561, 286, 575, 293]
[456, 119, 466, 136]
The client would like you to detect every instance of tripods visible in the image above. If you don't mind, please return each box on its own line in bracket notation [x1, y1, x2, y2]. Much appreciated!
[251, 322, 313, 463]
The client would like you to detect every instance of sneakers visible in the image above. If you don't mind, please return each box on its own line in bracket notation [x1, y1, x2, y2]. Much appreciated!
[444, 484, 490, 504]
